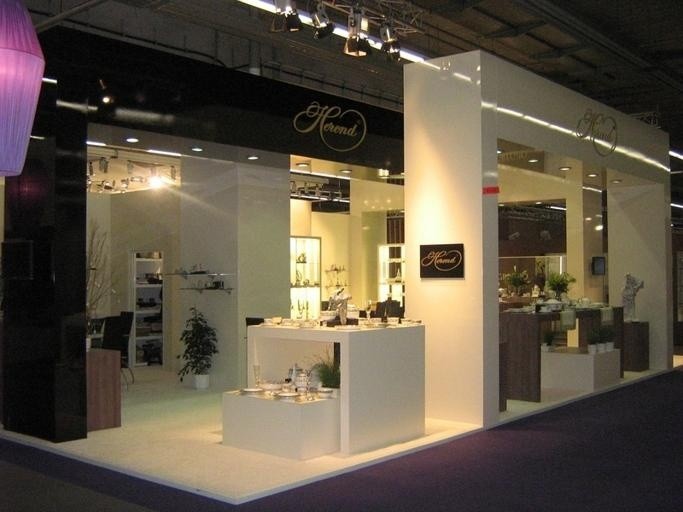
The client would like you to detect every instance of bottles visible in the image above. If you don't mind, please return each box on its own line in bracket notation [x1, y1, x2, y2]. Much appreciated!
[282, 379, 290, 393]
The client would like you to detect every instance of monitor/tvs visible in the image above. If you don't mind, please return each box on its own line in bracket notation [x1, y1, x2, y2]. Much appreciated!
[592, 257, 605, 276]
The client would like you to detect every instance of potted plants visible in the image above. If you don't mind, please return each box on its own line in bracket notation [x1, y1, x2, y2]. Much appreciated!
[308, 344, 340, 398]
[176, 305, 220, 391]
[540, 329, 556, 352]
[586, 325, 616, 355]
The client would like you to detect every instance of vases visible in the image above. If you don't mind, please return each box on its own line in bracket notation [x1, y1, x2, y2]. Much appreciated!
[86, 338, 92, 352]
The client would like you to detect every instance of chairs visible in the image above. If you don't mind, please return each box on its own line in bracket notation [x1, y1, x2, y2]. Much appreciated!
[103, 312, 134, 391]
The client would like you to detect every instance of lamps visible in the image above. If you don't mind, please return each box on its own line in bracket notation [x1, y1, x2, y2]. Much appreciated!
[270, 0, 422, 64]
[377, 169, 390, 180]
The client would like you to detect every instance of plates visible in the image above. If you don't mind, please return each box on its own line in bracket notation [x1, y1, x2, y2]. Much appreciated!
[278, 393, 300, 398]
[244, 388, 263, 393]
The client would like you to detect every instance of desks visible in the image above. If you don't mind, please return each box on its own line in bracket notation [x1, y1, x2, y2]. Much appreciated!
[503, 306, 623, 403]
[246, 320, 424, 458]
[223, 392, 340, 462]
[86, 348, 121, 433]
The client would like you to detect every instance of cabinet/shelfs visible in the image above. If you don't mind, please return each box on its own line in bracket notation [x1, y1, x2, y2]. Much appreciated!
[290, 235, 321, 320]
[377, 243, 404, 308]
[130, 249, 162, 367]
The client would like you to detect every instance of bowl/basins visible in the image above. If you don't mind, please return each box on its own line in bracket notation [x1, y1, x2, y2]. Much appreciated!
[318, 388, 333, 398]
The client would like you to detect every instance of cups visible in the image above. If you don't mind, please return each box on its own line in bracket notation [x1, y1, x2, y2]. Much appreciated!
[588, 342, 615, 354]
[263, 317, 423, 329]
